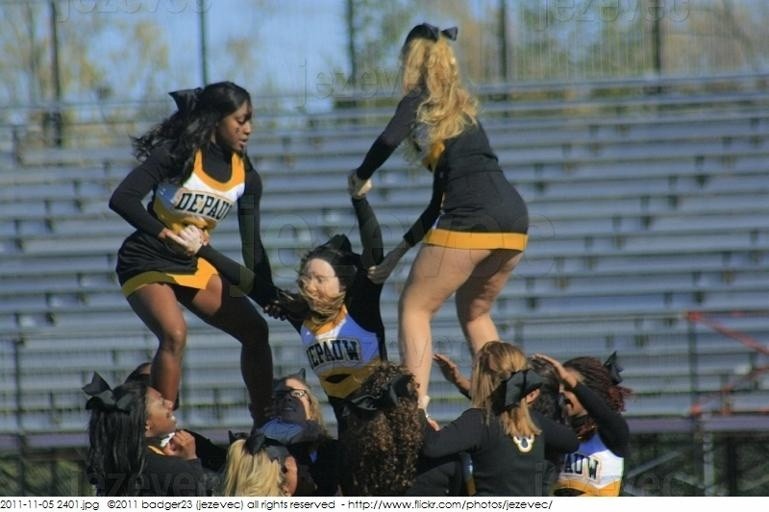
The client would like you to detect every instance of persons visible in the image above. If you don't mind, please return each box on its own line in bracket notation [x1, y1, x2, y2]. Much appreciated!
[348, 23, 529, 408]
[109, 82, 286, 436]
[85, 341, 629, 496]
[165, 176, 383, 426]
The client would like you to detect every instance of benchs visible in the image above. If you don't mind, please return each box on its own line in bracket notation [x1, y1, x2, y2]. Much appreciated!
[1, 70, 769, 448]
